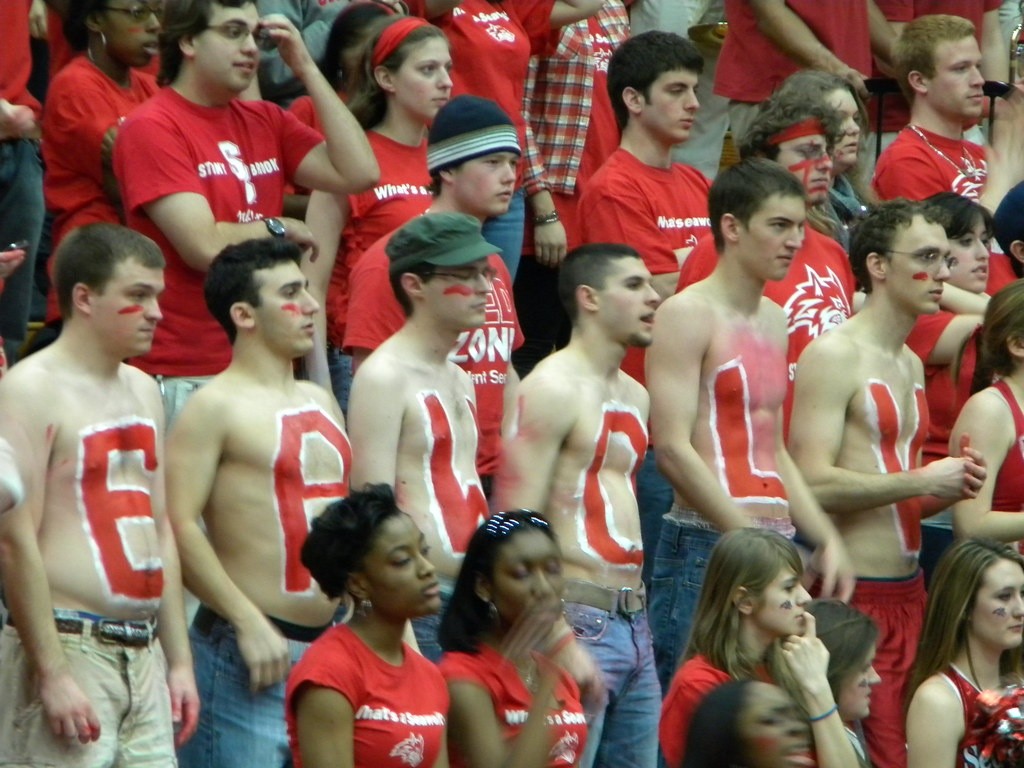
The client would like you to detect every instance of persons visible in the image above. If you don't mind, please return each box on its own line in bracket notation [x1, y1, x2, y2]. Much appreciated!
[346, 212, 493, 663]
[645, 157, 853, 701]
[682, 680, 815, 768]
[902, 537, 1024, 768]
[786, 198, 987, 768]
[492, 243, 662, 768]
[285, 482, 450, 768]
[801, 599, 882, 768]
[949, 276, 1024, 554]
[436, 510, 602, 768]
[657, 528, 859, 768]
[164, 239, 352, 768]
[0, 0, 1024, 589]
[0, 223, 200, 768]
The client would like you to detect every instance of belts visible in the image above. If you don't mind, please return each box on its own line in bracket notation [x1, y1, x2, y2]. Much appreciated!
[6, 613, 158, 646]
[561, 580, 647, 617]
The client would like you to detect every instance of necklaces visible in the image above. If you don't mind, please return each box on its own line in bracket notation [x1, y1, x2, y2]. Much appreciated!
[907, 124, 976, 178]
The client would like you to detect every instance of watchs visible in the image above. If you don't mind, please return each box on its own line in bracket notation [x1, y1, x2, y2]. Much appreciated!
[262, 216, 285, 241]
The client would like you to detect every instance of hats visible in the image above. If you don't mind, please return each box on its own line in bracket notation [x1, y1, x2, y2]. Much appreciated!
[426, 93, 523, 176]
[385, 212, 503, 275]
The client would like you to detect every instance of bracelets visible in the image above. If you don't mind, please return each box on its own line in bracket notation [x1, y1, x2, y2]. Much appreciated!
[534, 210, 559, 225]
[809, 703, 838, 721]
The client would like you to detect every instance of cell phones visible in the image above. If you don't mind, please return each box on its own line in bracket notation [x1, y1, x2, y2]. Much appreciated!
[1, 240, 30, 253]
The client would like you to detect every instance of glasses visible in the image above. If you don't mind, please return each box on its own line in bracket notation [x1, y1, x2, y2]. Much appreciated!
[872, 247, 958, 270]
[106, 4, 166, 21]
[204, 23, 270, 47]
[416, 267, 499, 282]
